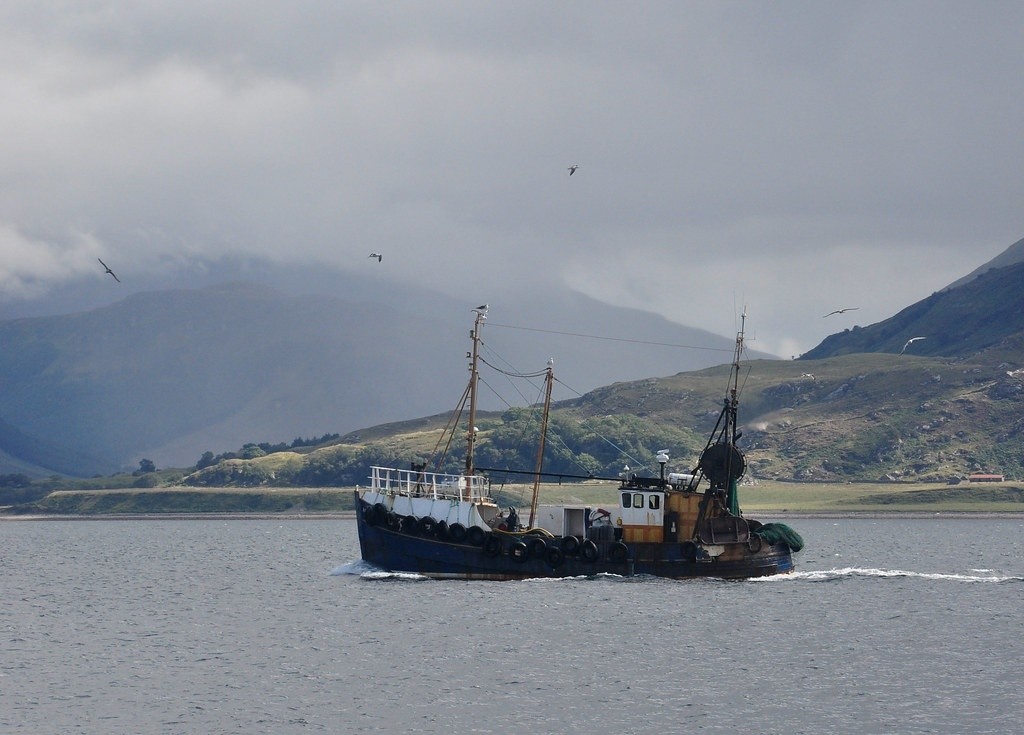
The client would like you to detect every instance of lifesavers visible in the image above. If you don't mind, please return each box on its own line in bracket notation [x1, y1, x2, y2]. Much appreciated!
[510, 534, 599, 566]
[607, 542, 629, 564]
[364, 503, 503, 556]
[681, 541, 697, 559]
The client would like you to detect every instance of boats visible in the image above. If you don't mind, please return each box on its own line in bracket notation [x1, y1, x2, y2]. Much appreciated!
[356, 304, 803, 579]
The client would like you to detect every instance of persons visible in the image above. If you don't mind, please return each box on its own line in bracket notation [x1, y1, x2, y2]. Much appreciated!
[507, 505, 520, 532]
